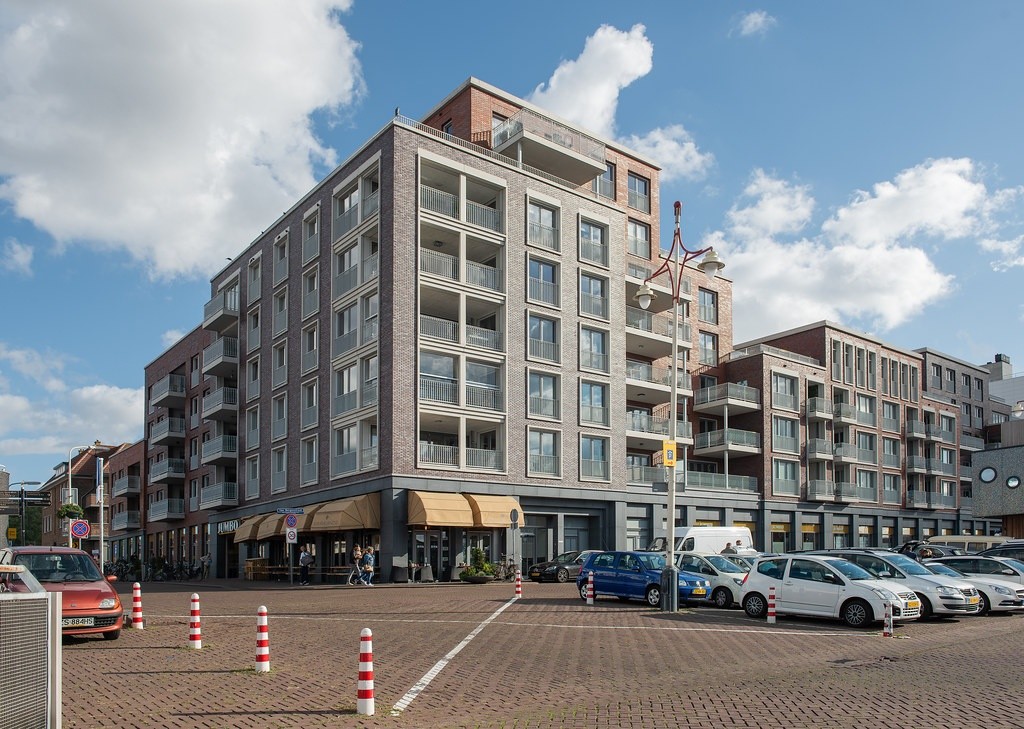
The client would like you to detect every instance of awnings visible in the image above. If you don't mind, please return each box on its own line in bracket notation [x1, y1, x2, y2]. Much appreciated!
[405, 490, 474, 528]
[462, 494, 525, 528]
[234, 492, 380, 544]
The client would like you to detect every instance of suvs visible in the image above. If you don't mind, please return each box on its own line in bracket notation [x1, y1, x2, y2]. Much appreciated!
[893, 534, 1024, 616]
[802, 547, 980, 623]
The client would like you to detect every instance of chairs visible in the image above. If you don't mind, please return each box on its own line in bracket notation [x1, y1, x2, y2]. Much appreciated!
[868, 561, 877, 574]
[642, 561, 651, 569]
[599, 559, 609, 567]
[768, 567, 782, 577]
[620, 559, 626, 567]
[683, 556, 697, 571]
[791, 565, 801, 574]
[50, 559, 73, 579]
[710, 559, 721, 570]
[658, 558, 665, 569]
[16, 560, 36, 579]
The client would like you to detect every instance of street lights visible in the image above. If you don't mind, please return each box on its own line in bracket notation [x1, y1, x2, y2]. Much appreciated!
[69, 445, 111, 548]
[9, 481, 42, 547]
[631, 201, 726, 613]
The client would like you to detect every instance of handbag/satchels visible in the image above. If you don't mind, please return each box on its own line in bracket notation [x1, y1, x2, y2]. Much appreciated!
[301, 553, 315, 567]
[364, 565, 372, 572]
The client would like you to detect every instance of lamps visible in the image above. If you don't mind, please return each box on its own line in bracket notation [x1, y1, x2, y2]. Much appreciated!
[433, 241, 443, 248]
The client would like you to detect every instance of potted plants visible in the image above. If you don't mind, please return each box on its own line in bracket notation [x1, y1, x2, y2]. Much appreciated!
[460, 547, 498, 584]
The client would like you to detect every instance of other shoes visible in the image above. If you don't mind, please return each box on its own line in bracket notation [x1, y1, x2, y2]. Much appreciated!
[300, 581, 309, 585]
[362, 582, 367, 585]
[346, 583, 353, 585]
[369, 583, 374, 586]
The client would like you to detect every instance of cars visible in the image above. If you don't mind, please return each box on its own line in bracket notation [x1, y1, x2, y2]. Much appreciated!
[716, 547, 814, 573]
[659, 551, 749, 608]
[526, 550, 614, 583]
[738, 553, 921, 628]
[0, 546, 124, 640]
[576, 550, 713, 608]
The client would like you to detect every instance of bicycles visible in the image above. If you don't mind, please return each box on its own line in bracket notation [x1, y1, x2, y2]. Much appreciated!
[491, 552, 516, 582]
[97, 561, 204, 582]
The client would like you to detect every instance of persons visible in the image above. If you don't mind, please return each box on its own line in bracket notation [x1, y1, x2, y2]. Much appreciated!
[903, 546, 936, 563]
[150, 553, 166, 572]
[299, 546, 312, 586]
[720, 540, 743, 565]
[200, 552, 212, 579]
[130, 552, 139, 570]
[346, 545, 375, 586]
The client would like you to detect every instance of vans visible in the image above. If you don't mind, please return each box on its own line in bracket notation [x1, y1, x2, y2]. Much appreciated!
[639, 526, 754, 569]
